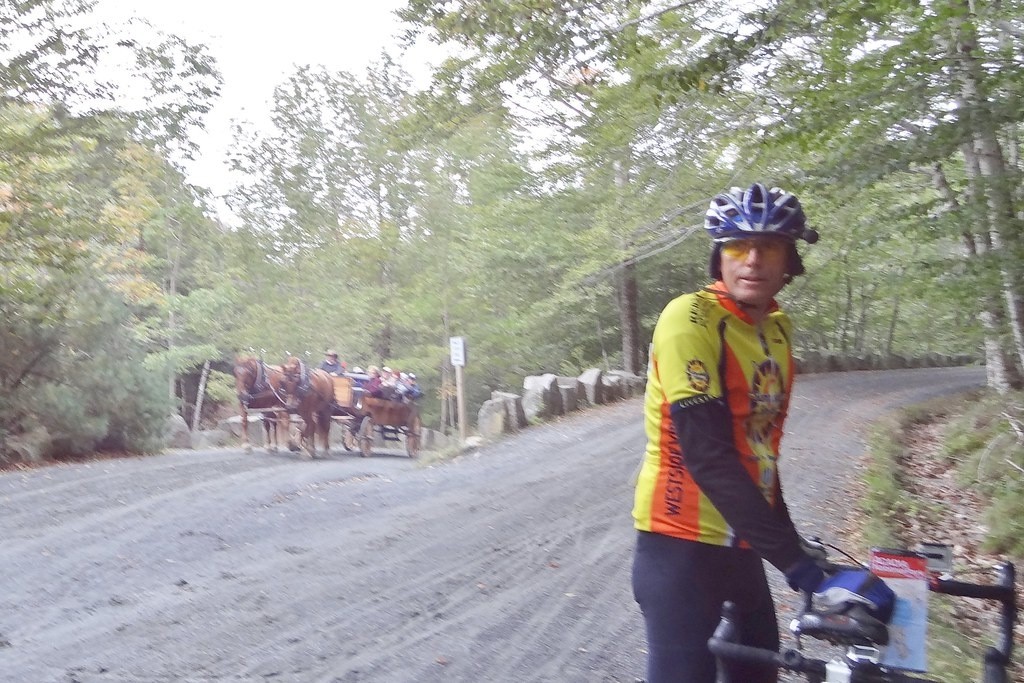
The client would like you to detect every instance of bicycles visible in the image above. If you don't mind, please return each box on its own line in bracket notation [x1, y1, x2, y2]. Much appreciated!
[708, 529, 1022, 683]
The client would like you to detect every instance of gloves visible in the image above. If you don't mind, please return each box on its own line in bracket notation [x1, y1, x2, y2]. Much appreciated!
[810, 566, 895, 623]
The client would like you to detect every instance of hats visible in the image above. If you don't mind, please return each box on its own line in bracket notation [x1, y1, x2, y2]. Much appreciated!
[409, 373, 416, 380]
[400, 372, 408, 380]
[323, 349, 338, 359]
[382, 366, 392, 373]
[367, 366, 382, 377]
[353, 367, 364, 374]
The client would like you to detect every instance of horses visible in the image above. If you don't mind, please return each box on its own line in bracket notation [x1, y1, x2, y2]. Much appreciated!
[232, 355, 334, 459]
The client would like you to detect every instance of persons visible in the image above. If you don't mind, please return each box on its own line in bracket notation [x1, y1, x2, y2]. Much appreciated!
[631, 183, 898, 682]
[348, 366, 424, 437]
[318, 349, 344, 377]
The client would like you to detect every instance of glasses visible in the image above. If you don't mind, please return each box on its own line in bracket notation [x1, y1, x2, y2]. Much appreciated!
[719, 239, 788, 260]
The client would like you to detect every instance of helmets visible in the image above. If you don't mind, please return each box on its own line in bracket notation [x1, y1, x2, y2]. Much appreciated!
[703, 184, 818, 244]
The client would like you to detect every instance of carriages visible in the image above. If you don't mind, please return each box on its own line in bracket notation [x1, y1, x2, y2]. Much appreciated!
[233, 345, 428, 462]
